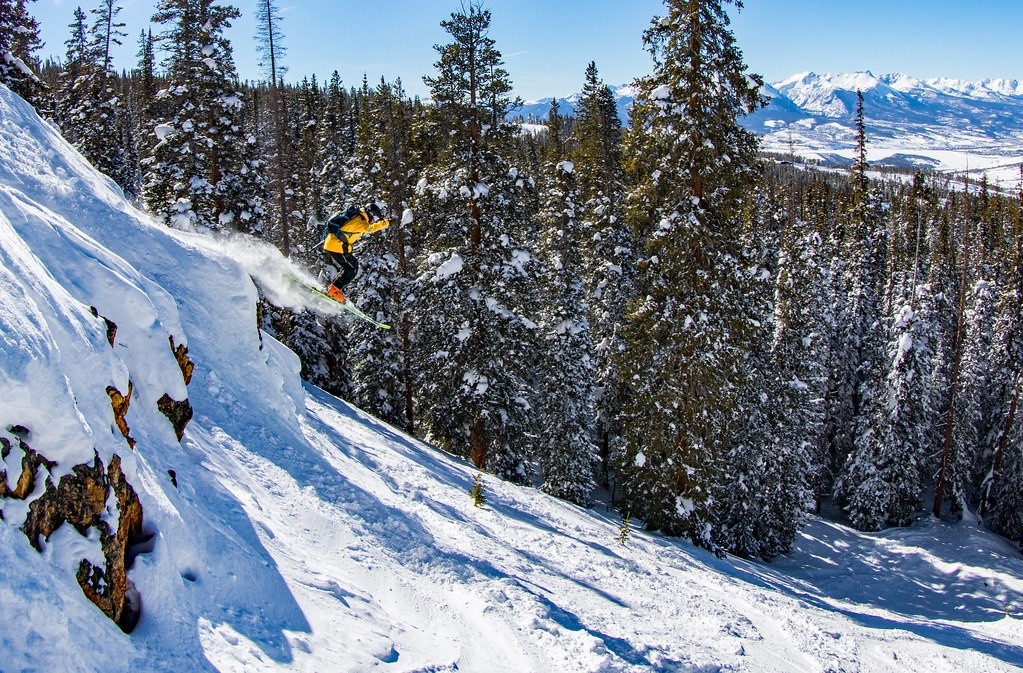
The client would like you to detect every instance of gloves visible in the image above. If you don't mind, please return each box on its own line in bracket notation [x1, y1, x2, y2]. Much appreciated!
[389, 218, 401, 226]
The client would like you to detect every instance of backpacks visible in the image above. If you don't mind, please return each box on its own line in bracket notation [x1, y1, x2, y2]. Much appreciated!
[327, 206, 360, 234]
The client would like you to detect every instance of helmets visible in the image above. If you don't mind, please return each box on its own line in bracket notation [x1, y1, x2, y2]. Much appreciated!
[365, 203, 381, 219]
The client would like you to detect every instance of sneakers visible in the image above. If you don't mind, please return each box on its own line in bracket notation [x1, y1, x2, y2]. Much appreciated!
[328, 284, 346, 302]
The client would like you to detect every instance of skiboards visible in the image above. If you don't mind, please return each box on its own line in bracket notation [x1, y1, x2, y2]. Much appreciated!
[284, 270, 392, 329]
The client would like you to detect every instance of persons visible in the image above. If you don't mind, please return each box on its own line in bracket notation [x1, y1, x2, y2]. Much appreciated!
[324, 203, 396, 302]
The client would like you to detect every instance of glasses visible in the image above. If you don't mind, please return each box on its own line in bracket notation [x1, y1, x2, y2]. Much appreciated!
[373, 215, 380, 221]
